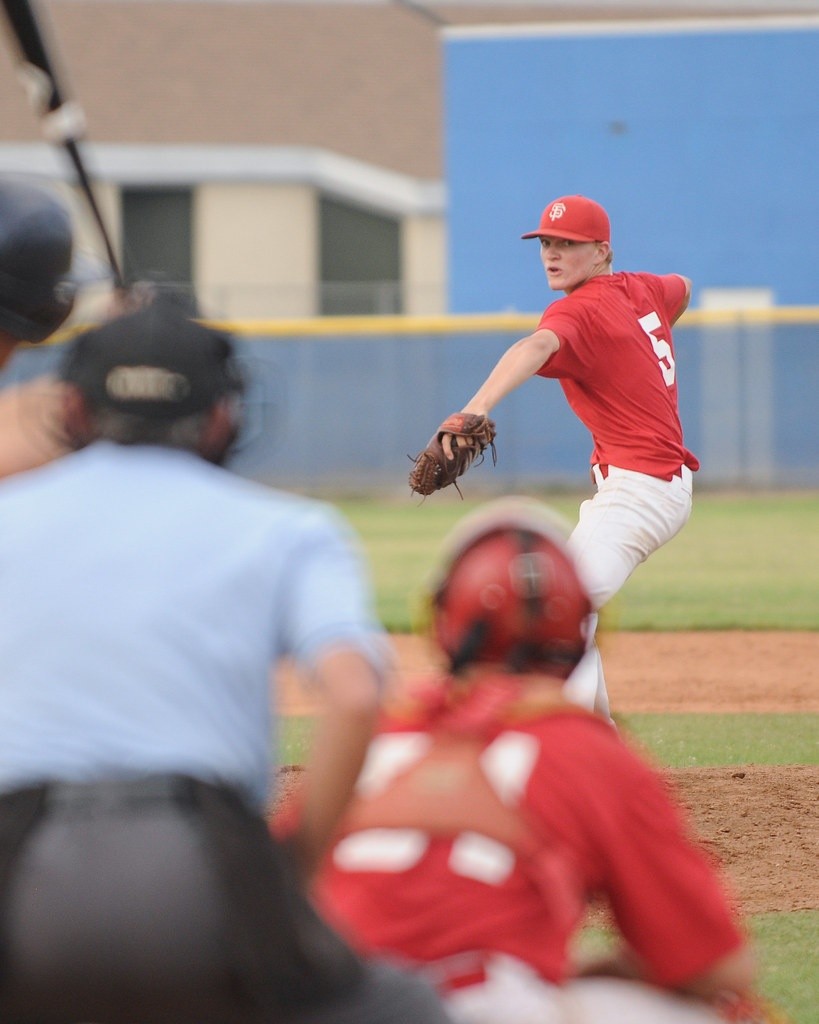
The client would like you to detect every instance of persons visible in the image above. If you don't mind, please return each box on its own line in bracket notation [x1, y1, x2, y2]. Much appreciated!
[410, 192, 700, 728]
[0, 172, 150, 486]
[1, 301, 390, 1024]
[274, 496, 787, 1024]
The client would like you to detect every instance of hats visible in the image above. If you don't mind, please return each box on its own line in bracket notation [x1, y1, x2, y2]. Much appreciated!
[0, 181, 83, 337]
[520, 193, 612, 244]
[60, 309, 247, 416]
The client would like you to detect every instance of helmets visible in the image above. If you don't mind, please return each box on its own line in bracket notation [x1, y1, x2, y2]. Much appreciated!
[426, 525, 598, 680]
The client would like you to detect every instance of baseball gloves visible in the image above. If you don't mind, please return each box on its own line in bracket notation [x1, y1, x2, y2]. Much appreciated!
[406, 412, 499, 508]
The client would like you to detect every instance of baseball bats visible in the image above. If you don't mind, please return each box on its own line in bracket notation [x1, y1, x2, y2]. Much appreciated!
[2, 1, 127, 291]
